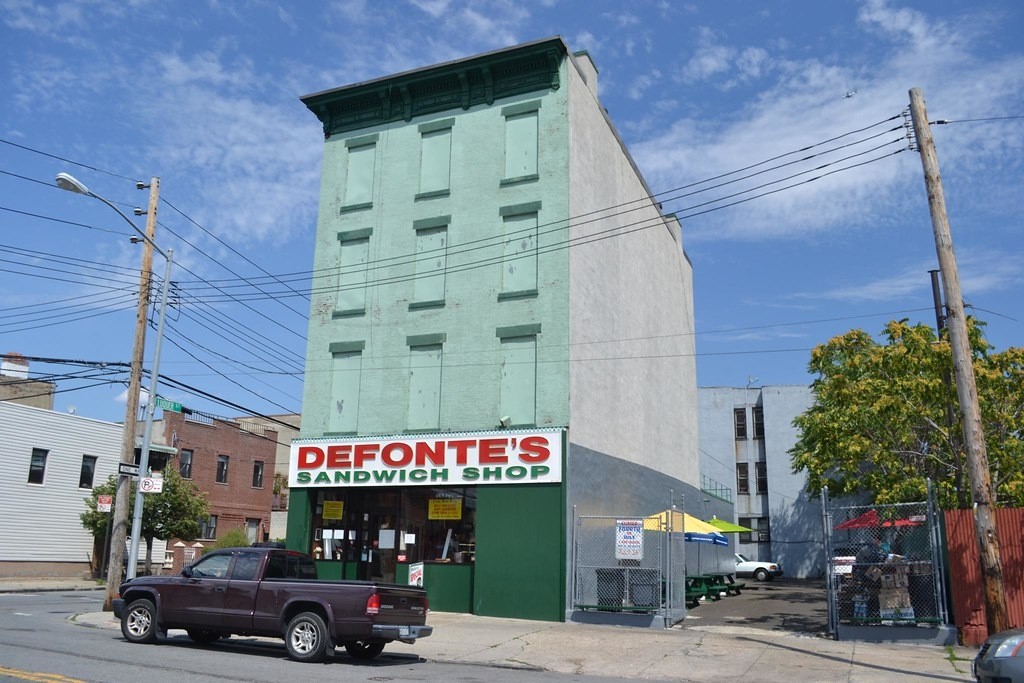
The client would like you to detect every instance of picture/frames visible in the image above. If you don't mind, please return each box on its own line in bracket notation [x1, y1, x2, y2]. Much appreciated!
[314, 528, 322, 541]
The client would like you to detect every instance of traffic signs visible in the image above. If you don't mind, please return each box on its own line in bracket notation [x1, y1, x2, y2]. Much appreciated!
[140, 478, 165, 494]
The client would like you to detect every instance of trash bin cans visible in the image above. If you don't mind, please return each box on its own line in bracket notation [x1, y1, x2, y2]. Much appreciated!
[595, 566, 628, 612]
[628, 566, 663, 604]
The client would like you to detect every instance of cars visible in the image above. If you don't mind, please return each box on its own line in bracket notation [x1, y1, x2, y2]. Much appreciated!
[970, 628, 1024, 683]
[734, 553, 783, 582]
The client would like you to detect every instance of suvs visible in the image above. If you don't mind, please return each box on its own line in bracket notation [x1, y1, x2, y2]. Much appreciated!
[827, 548, 861, 578]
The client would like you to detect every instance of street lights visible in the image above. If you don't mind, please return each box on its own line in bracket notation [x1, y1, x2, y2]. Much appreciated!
[54, 171, 175, 612]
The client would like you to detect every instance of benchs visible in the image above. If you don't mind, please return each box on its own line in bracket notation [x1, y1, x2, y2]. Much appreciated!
[662, 582, 745, 605]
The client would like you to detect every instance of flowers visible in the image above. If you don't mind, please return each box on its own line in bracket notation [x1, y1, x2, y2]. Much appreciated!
[313, 546, 323, 554]
[335, 548, 343, 554]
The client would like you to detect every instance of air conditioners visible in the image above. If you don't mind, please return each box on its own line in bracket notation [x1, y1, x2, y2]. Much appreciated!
[759, 534, 769, 541]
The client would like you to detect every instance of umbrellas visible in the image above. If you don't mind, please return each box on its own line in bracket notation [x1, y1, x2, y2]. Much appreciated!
[834, 508, 922, 530]
[706, 515, 756, 571]
[644, 505, 724, 532]
[670, 532, 728, 574]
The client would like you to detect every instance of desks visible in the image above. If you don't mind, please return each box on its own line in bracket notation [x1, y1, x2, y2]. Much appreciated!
[661, 578, 699, 607]
[703, 571, 742, 597]
[850, 573, 933, 619]
[686, 574, 720, 605]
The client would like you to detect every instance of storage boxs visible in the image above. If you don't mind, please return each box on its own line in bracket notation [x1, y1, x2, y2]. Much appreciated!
[841, 552, 932, 626]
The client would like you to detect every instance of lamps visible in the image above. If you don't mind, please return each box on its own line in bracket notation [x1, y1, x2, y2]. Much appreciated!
[501, 416, 511, 427]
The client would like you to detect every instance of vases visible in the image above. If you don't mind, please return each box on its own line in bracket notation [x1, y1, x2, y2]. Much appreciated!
[315, 553, 320, 559]
[337, 554, 340, 560]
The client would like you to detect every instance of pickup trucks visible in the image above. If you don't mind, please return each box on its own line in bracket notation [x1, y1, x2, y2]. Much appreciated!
[112, 542, 434, 664]
